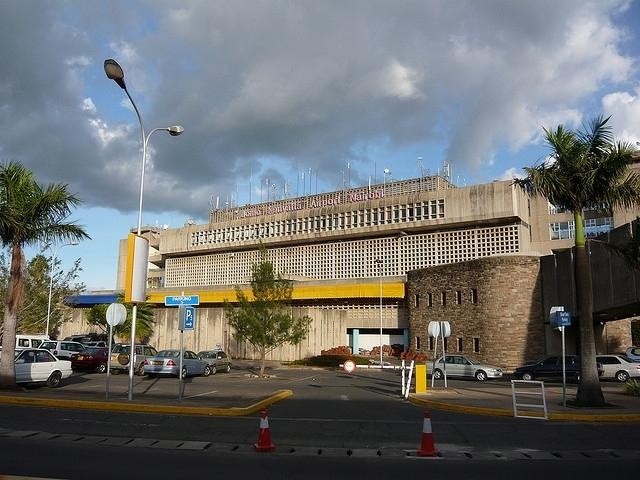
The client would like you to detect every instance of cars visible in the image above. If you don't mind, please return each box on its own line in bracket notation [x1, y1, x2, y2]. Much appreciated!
[139, 345, 212, 379]
[593, 353, 640, 383]
[0, 347, 74, 389]
[624, 346, 640, 360]
[422, 355, 506, 382]
[0, 331, 114, 374]
[510, 353, 606, 385]
[194, 346, 233, 375]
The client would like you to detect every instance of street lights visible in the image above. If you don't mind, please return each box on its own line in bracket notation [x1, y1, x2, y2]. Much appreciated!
[374, 257, 385, 365]
[100, 56, 188, 405]
[34, 238, 82, 336]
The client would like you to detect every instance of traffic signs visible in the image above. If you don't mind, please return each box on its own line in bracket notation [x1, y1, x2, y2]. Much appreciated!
[164, 294, 200, 308]
[184, 308, 195, 330]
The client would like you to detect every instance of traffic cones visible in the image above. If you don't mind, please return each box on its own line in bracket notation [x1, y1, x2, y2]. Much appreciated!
[250, 409, 278, 453]
[414, 410, 444, 457]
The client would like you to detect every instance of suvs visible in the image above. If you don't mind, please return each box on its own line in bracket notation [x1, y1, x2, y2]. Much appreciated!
[111, 342, 160, 377]
